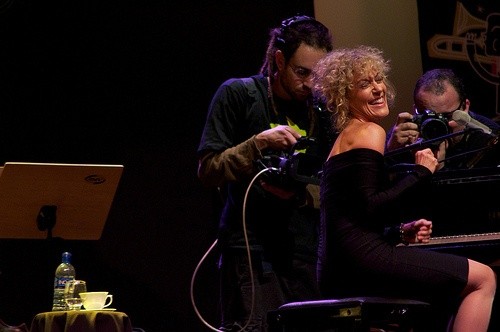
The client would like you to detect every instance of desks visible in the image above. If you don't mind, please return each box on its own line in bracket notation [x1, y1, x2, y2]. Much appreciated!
[32, 309, 132, 332]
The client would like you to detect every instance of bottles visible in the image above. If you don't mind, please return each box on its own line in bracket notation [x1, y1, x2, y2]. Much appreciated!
[51, 252, 76, 311]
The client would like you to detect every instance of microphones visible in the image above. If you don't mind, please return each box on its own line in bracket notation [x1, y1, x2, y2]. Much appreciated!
[453, 110, 497, 139]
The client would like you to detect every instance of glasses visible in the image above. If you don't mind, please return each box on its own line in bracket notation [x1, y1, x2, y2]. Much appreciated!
[444, 102, 463, 121]
[288, 60, 312, 78]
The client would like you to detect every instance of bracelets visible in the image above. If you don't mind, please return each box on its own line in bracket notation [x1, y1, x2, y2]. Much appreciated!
[400, 225, 405, 242]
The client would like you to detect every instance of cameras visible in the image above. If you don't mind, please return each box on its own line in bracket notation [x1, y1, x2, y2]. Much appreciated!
[406, 110, 456, 144]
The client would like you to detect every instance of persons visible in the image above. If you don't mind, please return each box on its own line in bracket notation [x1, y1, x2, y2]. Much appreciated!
[196, 16, 500, 332]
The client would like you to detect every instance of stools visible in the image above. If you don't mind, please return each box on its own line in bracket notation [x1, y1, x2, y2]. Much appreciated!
[276, 298, 433, 332]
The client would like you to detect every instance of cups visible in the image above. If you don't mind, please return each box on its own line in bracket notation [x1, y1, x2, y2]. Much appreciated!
[78, 291, 113, 309]
[64, 280, 87, 310]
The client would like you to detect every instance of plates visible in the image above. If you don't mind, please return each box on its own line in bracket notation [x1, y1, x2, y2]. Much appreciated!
[80, 307, 117, 311]
